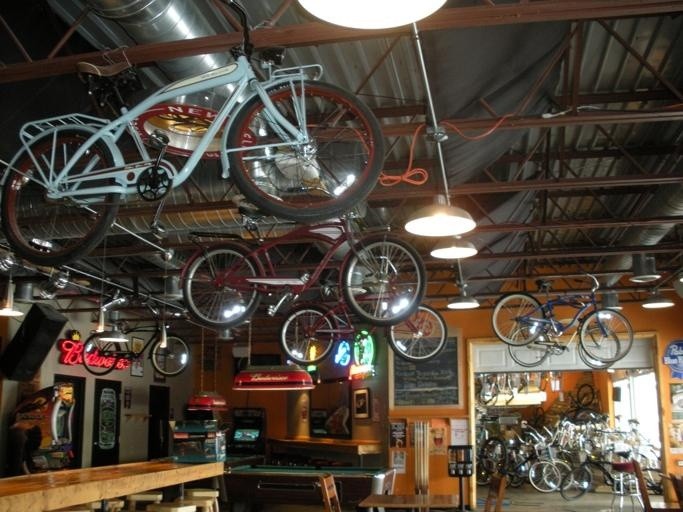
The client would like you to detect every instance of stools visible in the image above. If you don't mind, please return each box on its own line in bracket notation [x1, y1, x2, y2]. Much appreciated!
[58, 488, 222, 512]
[610, 462, 646, 510]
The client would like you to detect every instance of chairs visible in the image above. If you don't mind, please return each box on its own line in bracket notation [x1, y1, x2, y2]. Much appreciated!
[635, 460, 682, 512]
[321, 475, 344, 512]
[482, 474, 507, 511]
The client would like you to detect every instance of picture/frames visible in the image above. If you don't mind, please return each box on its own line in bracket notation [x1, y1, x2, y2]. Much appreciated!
[130, 335, 146, 378]
[352, 388, 372, 419]
[153, 344, 168, 383]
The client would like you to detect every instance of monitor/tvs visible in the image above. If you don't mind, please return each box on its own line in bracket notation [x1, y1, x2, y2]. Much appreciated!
[57, 415, 65, 437]
[234, 428, 260, 442]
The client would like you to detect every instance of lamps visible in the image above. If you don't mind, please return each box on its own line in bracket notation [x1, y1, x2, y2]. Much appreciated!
[1, 297, 24, 318]
[99, 310, 130, 345]
[403, 133, 481, 311]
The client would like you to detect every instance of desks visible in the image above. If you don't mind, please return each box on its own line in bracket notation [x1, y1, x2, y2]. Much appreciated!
[359, 494, 459, 509]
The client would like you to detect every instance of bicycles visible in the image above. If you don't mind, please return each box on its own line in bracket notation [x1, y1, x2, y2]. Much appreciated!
[0, 0, 387, 266]
[276, 271, 446, 364]
[490, 271, 634, 363]
[175, 184, 427, 333]
[473, 370, 662, 501]
[507, 289, 621, 371]
[80, 288, 189, 378]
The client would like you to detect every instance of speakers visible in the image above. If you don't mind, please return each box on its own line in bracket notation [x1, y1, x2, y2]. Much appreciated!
[1, 303, 69, 381]
